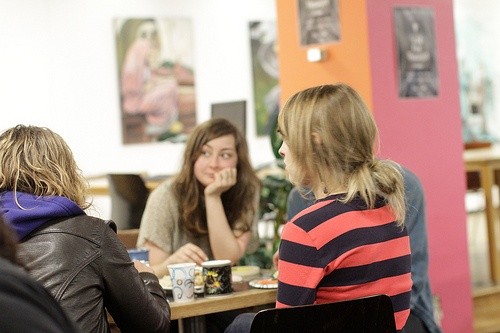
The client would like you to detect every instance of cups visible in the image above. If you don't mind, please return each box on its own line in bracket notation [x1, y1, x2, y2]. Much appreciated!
[166, 263, 196, 304]
[125, 248, 150, 263]
[200, 259, 232, 297]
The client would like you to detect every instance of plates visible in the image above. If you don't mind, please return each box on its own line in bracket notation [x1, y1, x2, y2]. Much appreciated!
[248, 279, 280, 288]
[160, 276, 236, 296]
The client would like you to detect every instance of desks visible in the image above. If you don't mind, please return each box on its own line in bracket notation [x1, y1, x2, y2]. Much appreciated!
[166, 268, 278, 333]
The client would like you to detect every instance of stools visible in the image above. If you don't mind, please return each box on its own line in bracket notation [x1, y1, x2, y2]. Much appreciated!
[465, 148, 500, 284]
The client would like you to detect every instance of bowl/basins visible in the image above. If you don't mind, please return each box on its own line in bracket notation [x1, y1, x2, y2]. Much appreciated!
[232, 266, 261, 282]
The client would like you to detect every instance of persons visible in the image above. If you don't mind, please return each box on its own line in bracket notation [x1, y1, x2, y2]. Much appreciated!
[136, 118, 261, 281]
[0, 124, 171, 333]
[222, 84, 443, 333]
